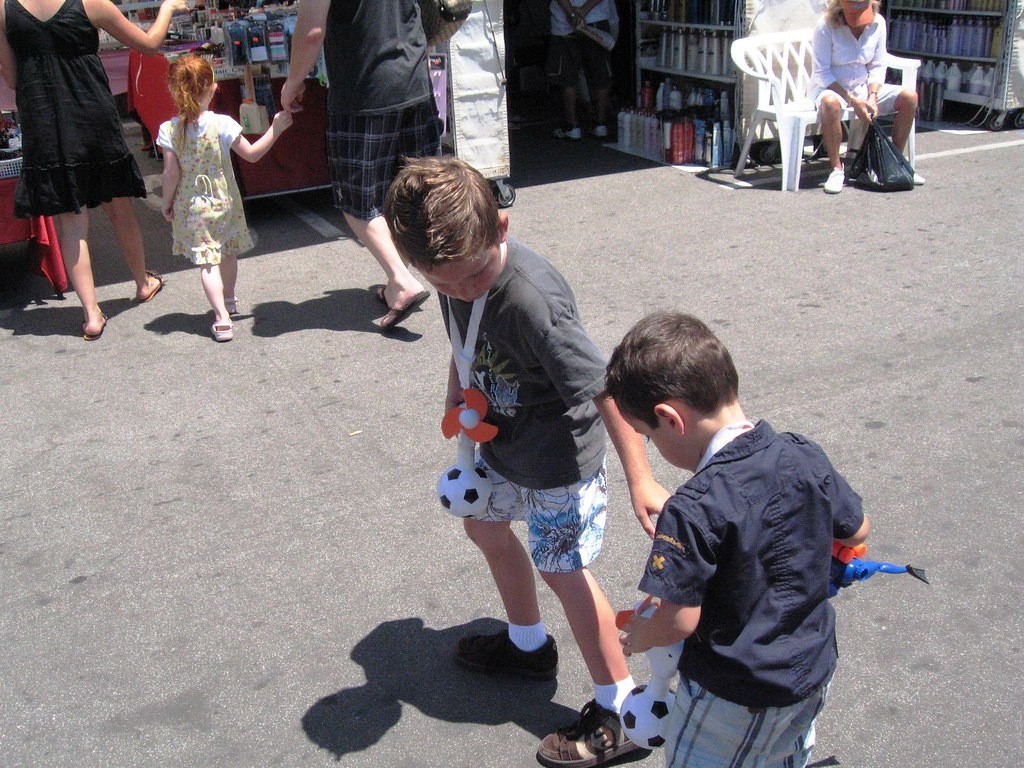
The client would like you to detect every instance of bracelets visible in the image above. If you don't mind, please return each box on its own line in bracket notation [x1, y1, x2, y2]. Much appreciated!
[849, 96, 853, 104]
[569, 12, 575, 17]
[871, 92, 877, 95]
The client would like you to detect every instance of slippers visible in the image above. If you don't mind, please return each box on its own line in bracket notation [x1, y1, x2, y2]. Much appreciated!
[380, 290, 430, 332]
[140, 268, 162, 302]
[82, 313, 108, 340]
[376, 286, 388, 305]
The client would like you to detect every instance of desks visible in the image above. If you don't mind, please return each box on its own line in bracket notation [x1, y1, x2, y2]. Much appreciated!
[0, 174, 68, 300]
[127, 42, 331, 203]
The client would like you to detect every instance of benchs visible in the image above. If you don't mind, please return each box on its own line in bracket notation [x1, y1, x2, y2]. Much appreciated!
[731, 29, 921, 191]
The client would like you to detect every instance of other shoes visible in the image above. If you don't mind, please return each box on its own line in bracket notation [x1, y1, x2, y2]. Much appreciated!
[508, 114, 526, 122]
[585, 126, 607, 136]
[554, 126, 582, 138]
[508, 122, 520, 130]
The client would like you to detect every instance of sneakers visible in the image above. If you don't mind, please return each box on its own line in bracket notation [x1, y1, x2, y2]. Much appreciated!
[455, 629, 559, 681]
[823, 162, 845, 193]
[913, 174, 926, 186]
[538, 697, 642, 768]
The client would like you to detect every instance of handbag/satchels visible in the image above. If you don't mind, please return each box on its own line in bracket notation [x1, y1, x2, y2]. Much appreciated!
[418, 0, 473, 46]
[845, 115, 914, 193]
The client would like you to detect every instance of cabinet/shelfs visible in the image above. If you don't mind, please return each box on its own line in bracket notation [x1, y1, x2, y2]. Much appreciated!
[426, 0, 515, 208]
[884, 0, 1024, 130]
[606, 0, 753, 173]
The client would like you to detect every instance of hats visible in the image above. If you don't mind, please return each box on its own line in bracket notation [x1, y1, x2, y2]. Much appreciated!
[840, 0, 874, 27]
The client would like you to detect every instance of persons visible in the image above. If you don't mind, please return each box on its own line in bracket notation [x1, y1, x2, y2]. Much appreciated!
[549, 0, 619, 141]
[812, 0, 924, 192]
[281, 0, 440, 330]
[0, 0, 189, 340]
[155, 56, 293, 342]
[383, 155, 670, 767]
[604, 314, 870, 768]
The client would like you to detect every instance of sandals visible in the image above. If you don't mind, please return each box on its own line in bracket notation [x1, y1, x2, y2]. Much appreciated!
[224, 296, 238, 313]
[211, 320, 233, 341]
